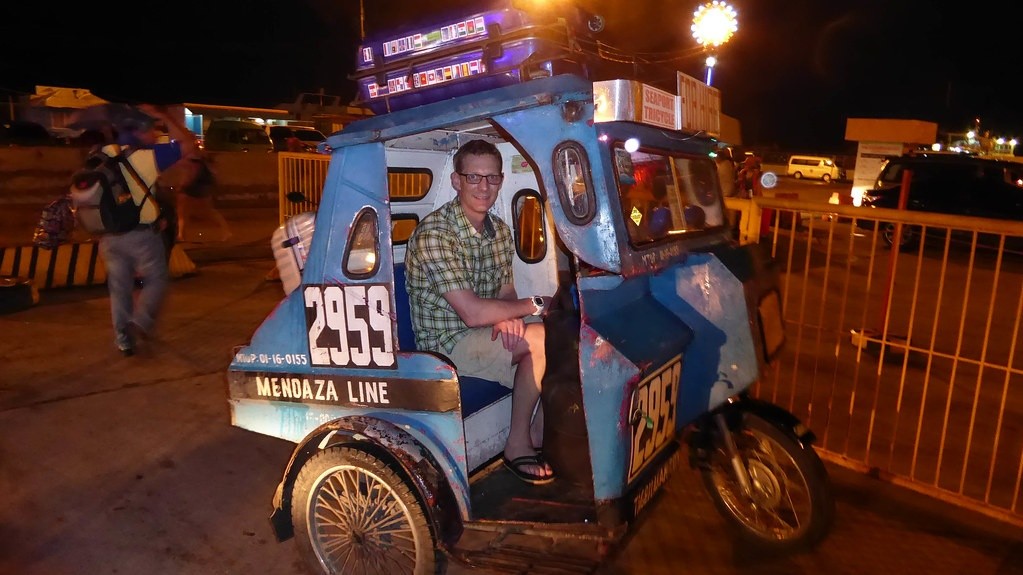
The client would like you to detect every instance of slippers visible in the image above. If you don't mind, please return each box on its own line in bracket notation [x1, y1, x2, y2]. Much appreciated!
[504, 451, 555, 485]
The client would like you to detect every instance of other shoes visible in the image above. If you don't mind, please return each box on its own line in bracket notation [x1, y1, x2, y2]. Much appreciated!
[119, 341, 136, 358]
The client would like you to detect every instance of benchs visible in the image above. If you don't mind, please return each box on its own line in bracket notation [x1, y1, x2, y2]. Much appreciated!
[365, 247, 544, 475]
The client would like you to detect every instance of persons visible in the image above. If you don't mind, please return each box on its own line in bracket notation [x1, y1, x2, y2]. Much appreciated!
[738, 155, 761, 197]
[85, 104, 195, 355]
[975, 129, 994, 153]
[404, 139, 555, 484]
[647, 179, 675, 233]
[714, 150, 738, 196]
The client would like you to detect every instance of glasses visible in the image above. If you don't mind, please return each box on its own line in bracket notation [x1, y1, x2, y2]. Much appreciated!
[460, 172, 504, 186]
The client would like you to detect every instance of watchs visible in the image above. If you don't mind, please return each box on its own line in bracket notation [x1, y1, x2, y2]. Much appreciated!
[528, 295, 545, 316]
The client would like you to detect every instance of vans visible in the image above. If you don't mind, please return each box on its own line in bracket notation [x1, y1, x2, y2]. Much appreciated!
[787, 155, 844, 183]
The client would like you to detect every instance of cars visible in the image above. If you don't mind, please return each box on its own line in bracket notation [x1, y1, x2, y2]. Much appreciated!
[201, 119, 274, 154]
[268, 125, 331, 156]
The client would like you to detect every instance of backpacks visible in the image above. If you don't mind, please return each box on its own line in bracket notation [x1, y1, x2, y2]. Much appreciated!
[89, 150, 155, 235]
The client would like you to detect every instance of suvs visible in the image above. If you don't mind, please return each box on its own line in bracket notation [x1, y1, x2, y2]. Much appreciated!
[855, 150, 1023, 259]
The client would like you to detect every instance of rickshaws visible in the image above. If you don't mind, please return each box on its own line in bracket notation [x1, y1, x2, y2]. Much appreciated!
[218, 0, 838, 575]
